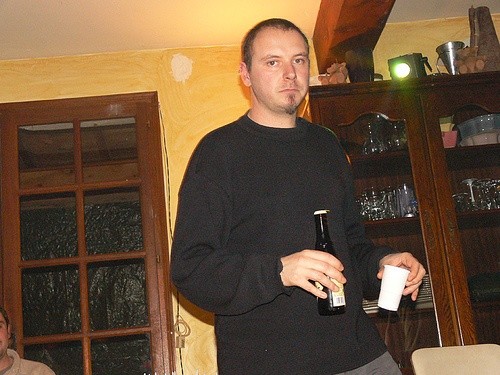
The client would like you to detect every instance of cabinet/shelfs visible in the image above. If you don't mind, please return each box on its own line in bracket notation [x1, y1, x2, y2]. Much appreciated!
[297, 71, 500, 375]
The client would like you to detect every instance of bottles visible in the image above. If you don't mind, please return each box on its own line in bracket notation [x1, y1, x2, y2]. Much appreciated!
[314, 210, 346, 316]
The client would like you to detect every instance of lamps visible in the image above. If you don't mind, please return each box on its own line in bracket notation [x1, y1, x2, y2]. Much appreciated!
[388, 53, 433, 80]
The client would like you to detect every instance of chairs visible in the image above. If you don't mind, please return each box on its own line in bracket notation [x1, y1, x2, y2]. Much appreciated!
[410, 344, 500, 375]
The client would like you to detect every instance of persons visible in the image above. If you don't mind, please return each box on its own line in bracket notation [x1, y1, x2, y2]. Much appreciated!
[0, 307, 57, 375]
[170, 18, 426, 375]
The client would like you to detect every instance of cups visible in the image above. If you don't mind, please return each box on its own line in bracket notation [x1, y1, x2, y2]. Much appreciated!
[452, 178, 500, 211]
[358, 183, 417, 220]
[362, 119, 407, 154]
[378, 265, 411, 311]
